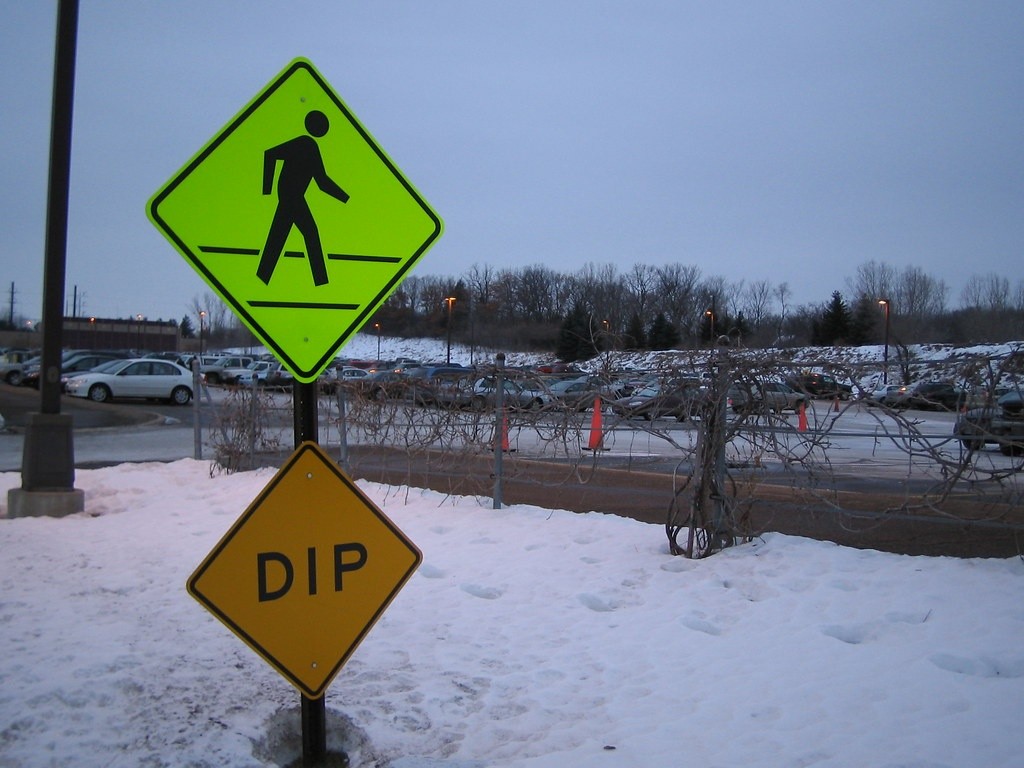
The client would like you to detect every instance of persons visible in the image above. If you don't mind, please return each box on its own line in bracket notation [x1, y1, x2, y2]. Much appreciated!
[177, 357, 186, 368]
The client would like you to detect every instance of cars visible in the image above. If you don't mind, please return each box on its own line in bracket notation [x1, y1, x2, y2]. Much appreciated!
[899, 379, 969, 411]
[868, 384, 914, 409]
[785, 372, 854, 401]
[0, 344, 718, 422]
[953, 386, 1024, 457]
[728, 379, 810, 415]
[64, 357, 207, 407]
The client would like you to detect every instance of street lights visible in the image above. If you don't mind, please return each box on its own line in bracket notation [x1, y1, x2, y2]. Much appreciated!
[706, 308, 713, 371]
[136, 313, 143, 358]
[604, 319, 610, 373]
[198, 311, 206, 355]
[372, 321, 382, 360]
[444, 296, 456, 363]
[877, 299, 889, 385]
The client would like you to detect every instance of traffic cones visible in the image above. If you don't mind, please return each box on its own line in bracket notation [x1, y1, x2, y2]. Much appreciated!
[488, 406, 517, 453]
[797, 403, 809, 431]
[580, 396, 612, 451]
[832, 399, 841, 412]
